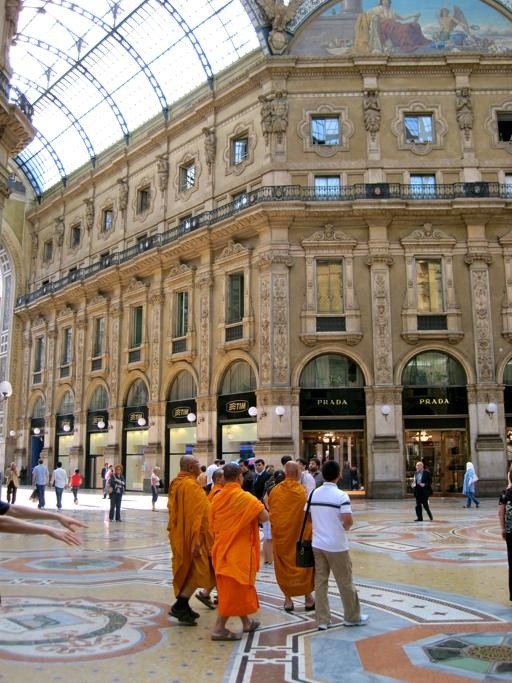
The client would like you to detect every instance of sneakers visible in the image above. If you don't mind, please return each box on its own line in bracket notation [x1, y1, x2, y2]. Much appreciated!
[318, 614, 369, 631]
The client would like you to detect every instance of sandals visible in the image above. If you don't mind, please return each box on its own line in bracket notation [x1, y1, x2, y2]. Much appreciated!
[169, 592, 218, 624]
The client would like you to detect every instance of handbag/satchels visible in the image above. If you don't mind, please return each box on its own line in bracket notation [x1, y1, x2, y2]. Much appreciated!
[296, 540, 314, 567]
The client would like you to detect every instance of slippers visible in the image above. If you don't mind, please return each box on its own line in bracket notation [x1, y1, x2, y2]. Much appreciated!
[243, 618, 260, 632]
[212, 629, 240, 640]
[284, 602, 293, 611]
[305, 601, 315, 610]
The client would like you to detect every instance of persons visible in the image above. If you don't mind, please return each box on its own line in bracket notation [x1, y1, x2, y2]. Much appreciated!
[206, 462, 269, 641]
[366, 0, 431, 50]
[498, 460, 512, 602]
[461, 461, 480, 508]
[4, 460, 20, 503]
[196, 454, 326, 565]
[151, 466, 161, 511]
[50, 460, 69, 509]
[101, 462, 109, 498]
[268, 459, 316, 612]
[167, 453, 210, 626]
[30, 457, 50, 509]
[107, 463, 127, 522]
[68, 467, 85, 503]
[303, 459, 370, 631]
[409, 460, 434, 521]
[194, 466, 227, 609]
[105, 464, 114, 500]
[0, 499, 90, 547]
[431, 6, 469, 48]
